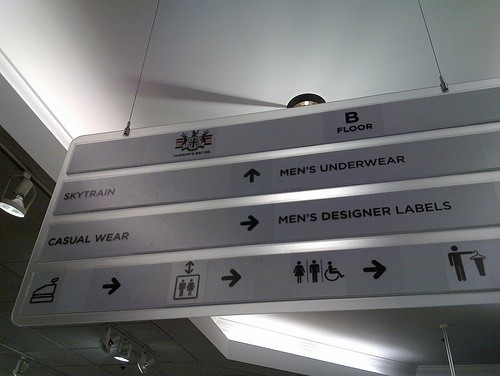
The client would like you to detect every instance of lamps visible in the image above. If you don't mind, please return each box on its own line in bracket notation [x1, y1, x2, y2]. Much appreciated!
[13, 357, 27, 376]
[1, 176, 37, 217]
[97, 327, 156, 375]
